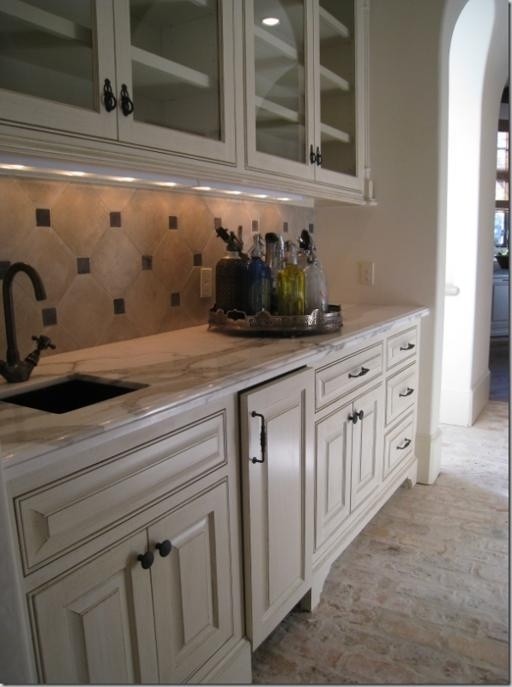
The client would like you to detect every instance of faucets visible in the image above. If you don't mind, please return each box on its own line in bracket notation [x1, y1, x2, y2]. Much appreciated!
[3, 262, 46, 363]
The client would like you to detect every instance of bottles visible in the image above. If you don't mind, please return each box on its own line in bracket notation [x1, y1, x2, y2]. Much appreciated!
[214, 227, 327, 314]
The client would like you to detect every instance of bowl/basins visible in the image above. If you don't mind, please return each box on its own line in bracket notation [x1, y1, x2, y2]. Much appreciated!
[495, 255, 508, 268]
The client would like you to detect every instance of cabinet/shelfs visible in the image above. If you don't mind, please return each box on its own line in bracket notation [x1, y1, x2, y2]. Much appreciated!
[314, 324, 420, 555]
[2, 1, 243, 192]
[238, 367, 311, 652]
[242, 1, 365, 192]
[11, 407, 240, 685]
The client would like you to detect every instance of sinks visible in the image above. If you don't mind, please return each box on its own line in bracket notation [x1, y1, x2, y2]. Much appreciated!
[0, 373, 151, 414]
